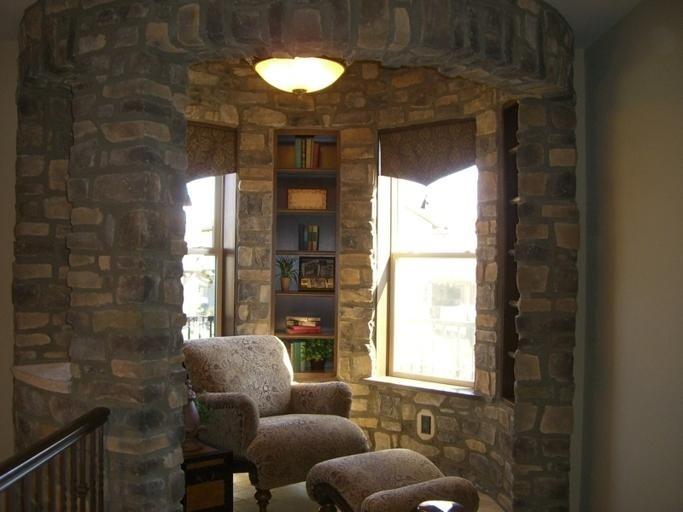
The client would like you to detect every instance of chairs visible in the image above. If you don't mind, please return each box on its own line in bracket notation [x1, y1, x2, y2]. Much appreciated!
[182, 335, 370, 511]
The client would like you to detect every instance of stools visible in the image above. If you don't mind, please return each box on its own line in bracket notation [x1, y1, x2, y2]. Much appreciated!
[305, 448, 479, 511]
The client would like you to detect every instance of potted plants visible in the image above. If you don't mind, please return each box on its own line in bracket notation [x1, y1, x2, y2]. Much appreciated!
[273, 256, 297, 292]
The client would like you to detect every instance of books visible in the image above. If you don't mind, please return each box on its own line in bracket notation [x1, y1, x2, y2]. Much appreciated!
[303, 224, 307, 250]
[294, 137, 319, 168]
[284, 315, 321, 372]
[311, 225, 318, 250]
[307, 225, 312, 251]
[298, 222, 304, 250]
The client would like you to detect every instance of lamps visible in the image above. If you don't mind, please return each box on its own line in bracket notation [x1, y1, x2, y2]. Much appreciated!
[255, 58, 347, 99]
